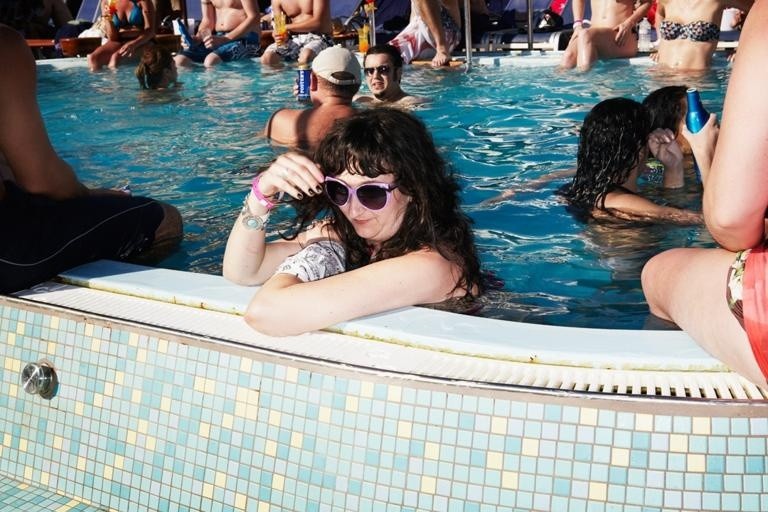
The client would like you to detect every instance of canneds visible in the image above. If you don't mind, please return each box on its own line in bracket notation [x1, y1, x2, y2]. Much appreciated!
[297, 64, 312, 100]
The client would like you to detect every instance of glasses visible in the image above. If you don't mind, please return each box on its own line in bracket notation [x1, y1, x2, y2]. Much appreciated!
[324, 176, 402, 212]
[364, 65, 390, 76]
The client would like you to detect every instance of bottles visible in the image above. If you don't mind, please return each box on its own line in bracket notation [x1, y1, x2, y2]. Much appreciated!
[176, 16, 196, 48]
[685, 87, 711, 186]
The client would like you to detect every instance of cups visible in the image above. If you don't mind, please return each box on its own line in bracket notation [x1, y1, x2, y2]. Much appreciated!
[273, 14, 286, 36]
[199, 28, 212, 40]
[357, 28, 369, 53]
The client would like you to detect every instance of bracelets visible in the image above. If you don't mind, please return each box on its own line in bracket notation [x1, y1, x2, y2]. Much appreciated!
[253, 172, 284, 208]
[572, 19, 582, 24]
[239, 197, 270, 230]
[572, 22, 582, 29]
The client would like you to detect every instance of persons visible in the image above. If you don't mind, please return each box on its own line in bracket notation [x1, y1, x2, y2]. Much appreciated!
[470, 86, 703, 214]
[552, 0, 652, 77]
[639, 0, 767, 389]
[1, 23, 183, 296]
[642, 0, 758, 82]
[542, 98, 706, 259]
[80, 1, 490, 158]
[222, 106, 504, 339]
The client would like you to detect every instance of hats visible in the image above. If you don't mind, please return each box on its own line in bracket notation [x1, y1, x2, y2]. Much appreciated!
[311, 44, 361, 85]
